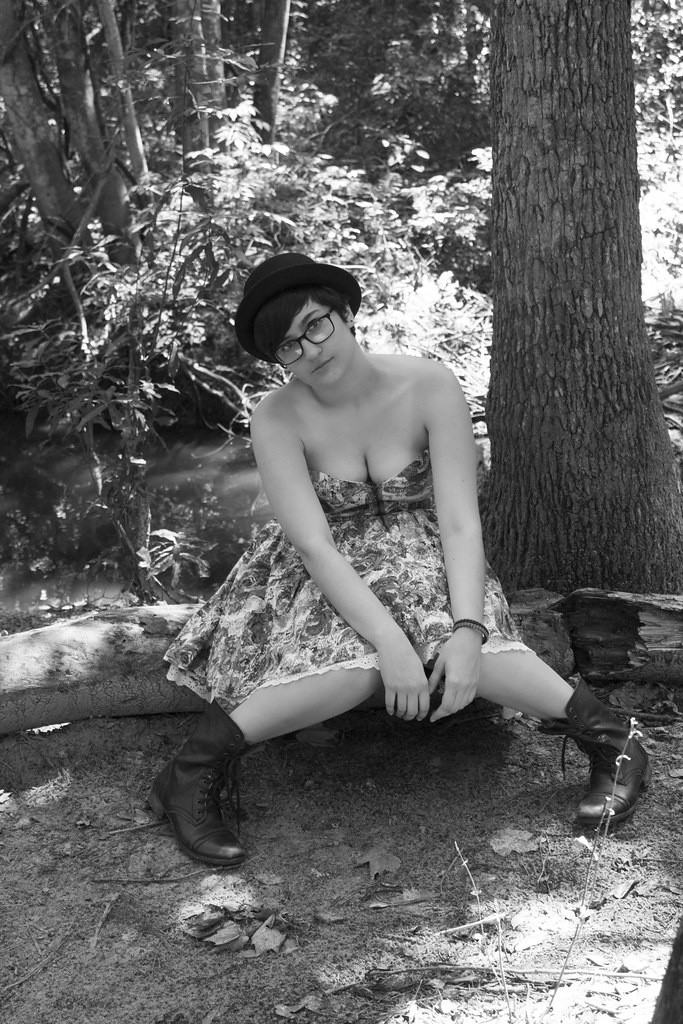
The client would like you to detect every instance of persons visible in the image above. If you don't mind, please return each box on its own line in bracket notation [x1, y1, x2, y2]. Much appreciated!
[145, 254, 652, 866]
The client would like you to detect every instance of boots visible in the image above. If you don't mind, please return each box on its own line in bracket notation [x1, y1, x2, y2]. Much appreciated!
[146, 697, 261, 866]
[538, 672, 651, 825]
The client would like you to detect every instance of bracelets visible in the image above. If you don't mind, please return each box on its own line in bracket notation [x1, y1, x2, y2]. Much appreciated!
[453, 619, 489, 645]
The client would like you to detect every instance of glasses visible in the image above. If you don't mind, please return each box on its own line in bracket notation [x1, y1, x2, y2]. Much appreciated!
[272, 307, 335, 366]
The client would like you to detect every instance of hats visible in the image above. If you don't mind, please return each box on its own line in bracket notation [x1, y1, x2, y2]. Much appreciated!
[235, 253, 361, 364]
